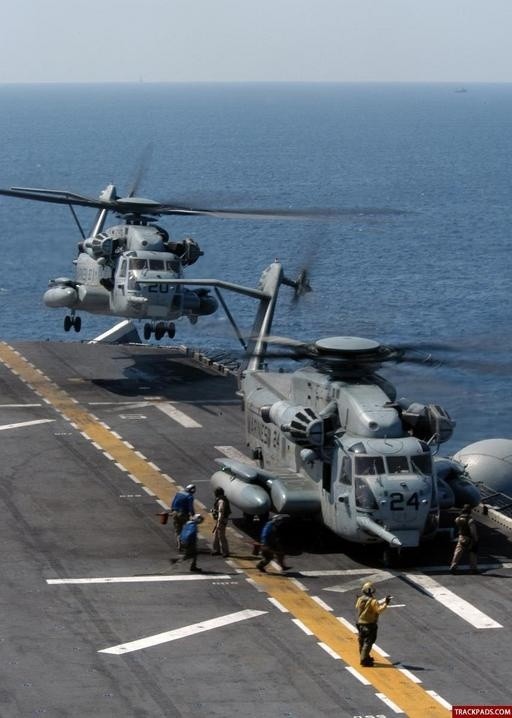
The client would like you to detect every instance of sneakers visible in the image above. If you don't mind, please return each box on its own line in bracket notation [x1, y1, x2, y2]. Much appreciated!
[190, 550, 294, 574]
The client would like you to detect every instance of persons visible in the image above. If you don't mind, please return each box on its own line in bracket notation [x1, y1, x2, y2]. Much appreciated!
[253, 515, 293, 574]
[212, 484, 234, 559]
[354, 581, 391, 667]
[447, 503, 479, 572]
[168, 514, 204, 572]
[154, 484, 198, 548]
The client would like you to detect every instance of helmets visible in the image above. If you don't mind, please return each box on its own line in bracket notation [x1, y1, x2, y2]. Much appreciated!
[185, 483, 196, 494]
[214, 486, 224, 497]
[272, 514, 283, 521]
[192, 513, 203, 524]
[359, 580, 377, 595]
